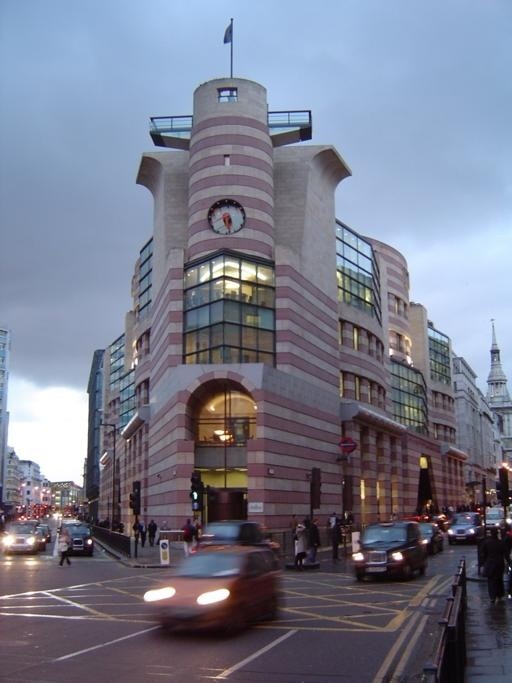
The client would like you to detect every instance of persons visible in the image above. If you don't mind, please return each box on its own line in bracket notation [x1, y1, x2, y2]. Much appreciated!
[58, 528, 73, 566]
[181, 519, 198, 558]
[293, 503, 511, 601]
[78, 511, 158, 547]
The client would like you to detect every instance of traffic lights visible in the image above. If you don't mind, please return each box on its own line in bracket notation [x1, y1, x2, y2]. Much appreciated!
[495, 467, 509, 506]
[190, 471, 204, 511]
[129, 480, 142, 516]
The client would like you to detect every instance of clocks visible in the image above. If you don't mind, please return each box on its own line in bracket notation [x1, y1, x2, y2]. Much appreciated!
[208, 198, 245, 234]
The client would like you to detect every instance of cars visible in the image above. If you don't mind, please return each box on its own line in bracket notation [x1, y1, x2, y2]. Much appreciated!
[141, 543, 284, 638]
[189, 520, 277, 558]
[419, 521, 446, 556]
[28, 509, 82, 553]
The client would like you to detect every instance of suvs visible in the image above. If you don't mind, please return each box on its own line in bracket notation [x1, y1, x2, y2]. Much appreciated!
[446, 510, 483, 544]
[3, 519, 40, 555]
[56, 521, 96, 556]
[350, 519, 430, 583]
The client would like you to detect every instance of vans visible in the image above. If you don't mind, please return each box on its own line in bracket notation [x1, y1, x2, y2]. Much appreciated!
[482, 506, 505, 531]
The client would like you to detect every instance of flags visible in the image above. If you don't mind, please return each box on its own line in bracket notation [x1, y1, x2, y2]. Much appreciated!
[224, 23, 233, 44]
